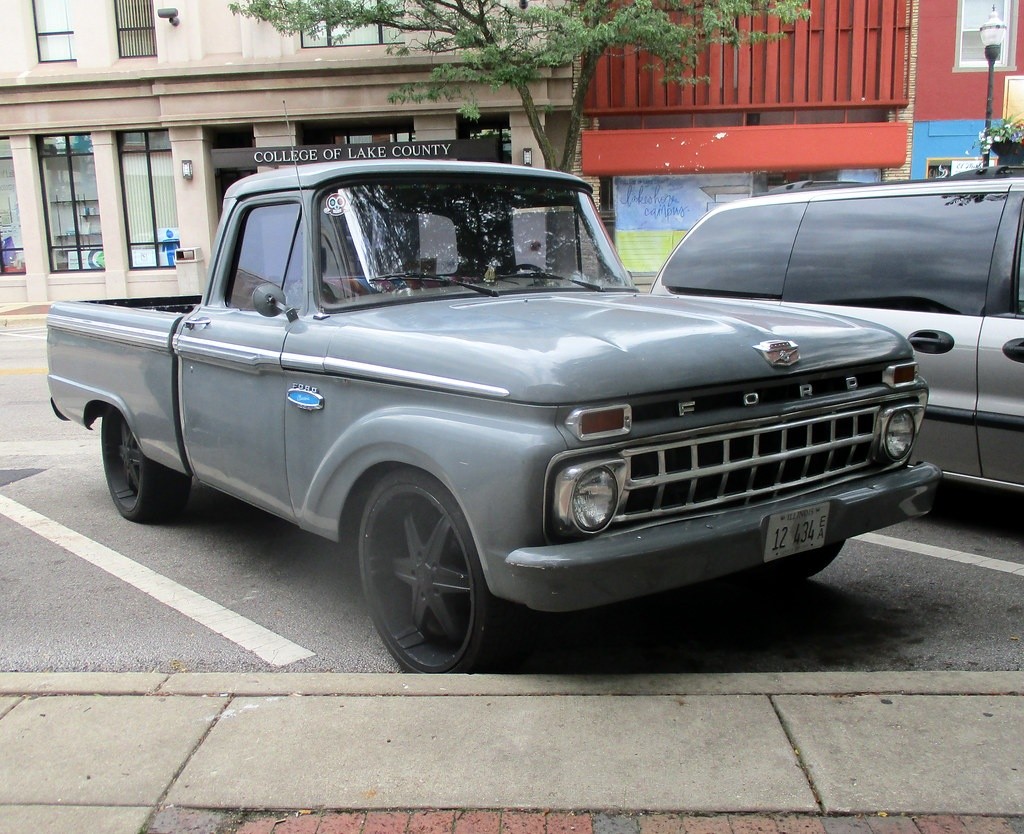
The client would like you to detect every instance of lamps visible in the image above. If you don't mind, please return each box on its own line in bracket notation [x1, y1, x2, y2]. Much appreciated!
[182, 159, 192, 179]
[523, 148, 532, 165]
[157, 8, 179, 27]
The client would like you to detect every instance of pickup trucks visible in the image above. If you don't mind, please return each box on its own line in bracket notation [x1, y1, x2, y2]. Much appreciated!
[47, 137, 942, 677]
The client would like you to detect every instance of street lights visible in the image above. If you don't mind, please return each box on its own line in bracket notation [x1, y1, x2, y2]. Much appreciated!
[979, 2, 1009, 167]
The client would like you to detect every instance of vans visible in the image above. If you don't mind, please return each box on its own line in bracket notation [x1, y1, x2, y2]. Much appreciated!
[651, 167, 1024, 496]
